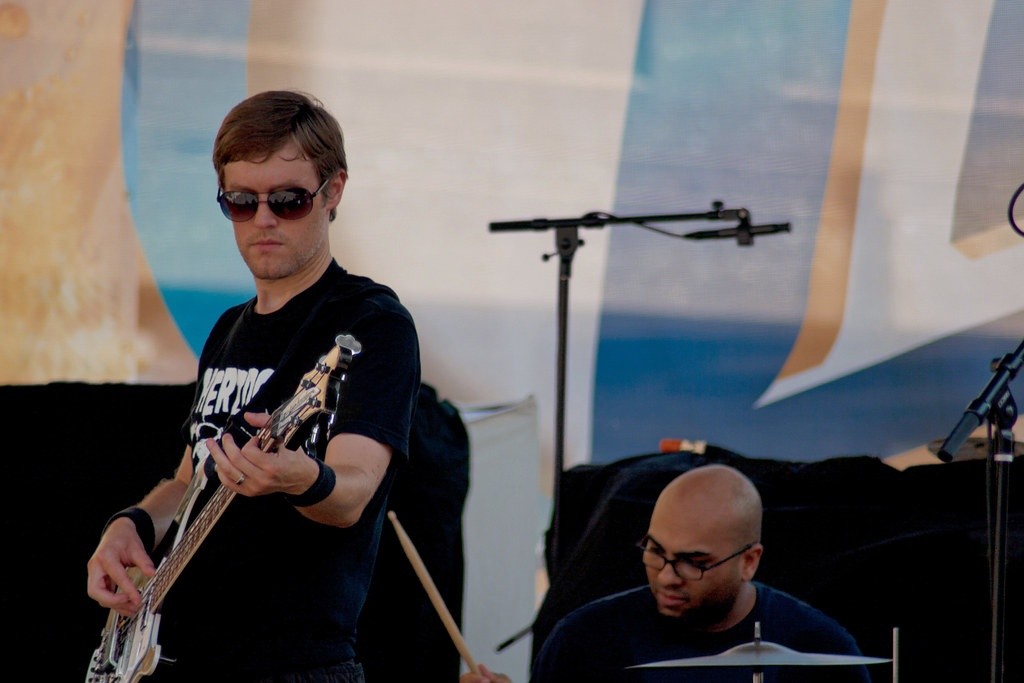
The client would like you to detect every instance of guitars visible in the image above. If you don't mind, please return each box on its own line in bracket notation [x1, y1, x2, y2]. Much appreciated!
[85, 333, 362, 683]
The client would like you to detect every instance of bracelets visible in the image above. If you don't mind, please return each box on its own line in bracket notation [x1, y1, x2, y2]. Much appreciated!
[101, 508, 155, 556]
[281, 459, 337, 507]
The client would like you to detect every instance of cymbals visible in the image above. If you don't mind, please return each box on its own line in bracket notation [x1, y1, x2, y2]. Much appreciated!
[624, 642, 893, 669]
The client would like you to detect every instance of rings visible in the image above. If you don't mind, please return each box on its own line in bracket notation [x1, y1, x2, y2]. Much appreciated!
[235, 473, 246, 486]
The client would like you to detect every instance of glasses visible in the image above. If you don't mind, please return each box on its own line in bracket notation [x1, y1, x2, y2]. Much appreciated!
[216, 178, 330, 223]
[635, 535, 753, 580]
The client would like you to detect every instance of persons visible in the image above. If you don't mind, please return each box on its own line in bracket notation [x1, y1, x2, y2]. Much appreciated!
[458, 464, 873, 683]
[87, 85, 421, 682]
